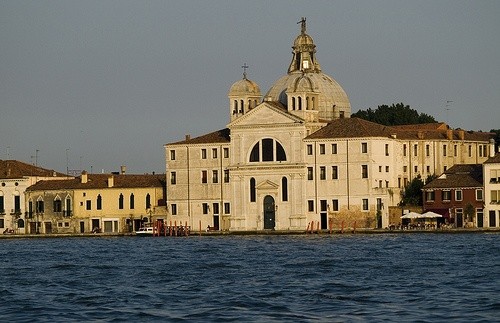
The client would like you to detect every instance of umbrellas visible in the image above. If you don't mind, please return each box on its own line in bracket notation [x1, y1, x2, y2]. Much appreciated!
[400, 212, 442, 218]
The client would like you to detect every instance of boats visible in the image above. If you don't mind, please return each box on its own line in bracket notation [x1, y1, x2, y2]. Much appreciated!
[135, 227, 159, 236]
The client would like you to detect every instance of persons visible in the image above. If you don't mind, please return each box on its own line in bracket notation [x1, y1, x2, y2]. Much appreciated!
[389, 222, 451, 230]
[297, 16, 307, 31]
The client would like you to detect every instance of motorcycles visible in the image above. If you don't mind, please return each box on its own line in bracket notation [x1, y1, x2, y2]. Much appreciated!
[3, 227, 15, 235]
[91, 227, 102, 233]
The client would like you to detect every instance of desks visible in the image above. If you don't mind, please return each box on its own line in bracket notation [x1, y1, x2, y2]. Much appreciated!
[390, 224, 435, 230]
